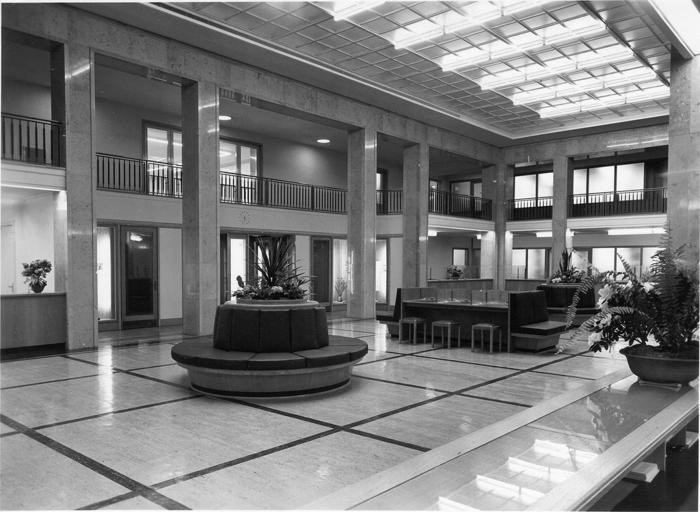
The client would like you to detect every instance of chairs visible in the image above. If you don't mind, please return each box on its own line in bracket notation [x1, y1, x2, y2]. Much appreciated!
[373, 276, 605, 360]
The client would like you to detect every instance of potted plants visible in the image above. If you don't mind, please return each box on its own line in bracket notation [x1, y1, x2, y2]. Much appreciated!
[543, 212, 699, 393]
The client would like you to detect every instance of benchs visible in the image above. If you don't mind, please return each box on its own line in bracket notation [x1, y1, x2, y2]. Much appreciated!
[165, 306, 373, 403]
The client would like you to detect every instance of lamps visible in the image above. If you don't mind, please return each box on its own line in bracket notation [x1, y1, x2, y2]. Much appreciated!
[333, 1, 386, 21]
[604, 227, 668, 236]
[480, 44, 670, 119]
[394, 0, 544, 50]
[441, 18, 608, 72]
[534, 230, 577, 238]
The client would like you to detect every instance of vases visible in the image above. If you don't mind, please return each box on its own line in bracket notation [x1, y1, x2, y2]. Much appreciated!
[28, 274, 47, 294]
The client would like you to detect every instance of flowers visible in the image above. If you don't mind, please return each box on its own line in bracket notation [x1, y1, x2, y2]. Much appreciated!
[20, 258, 55, 287]
[227, 229, 320, 306]
